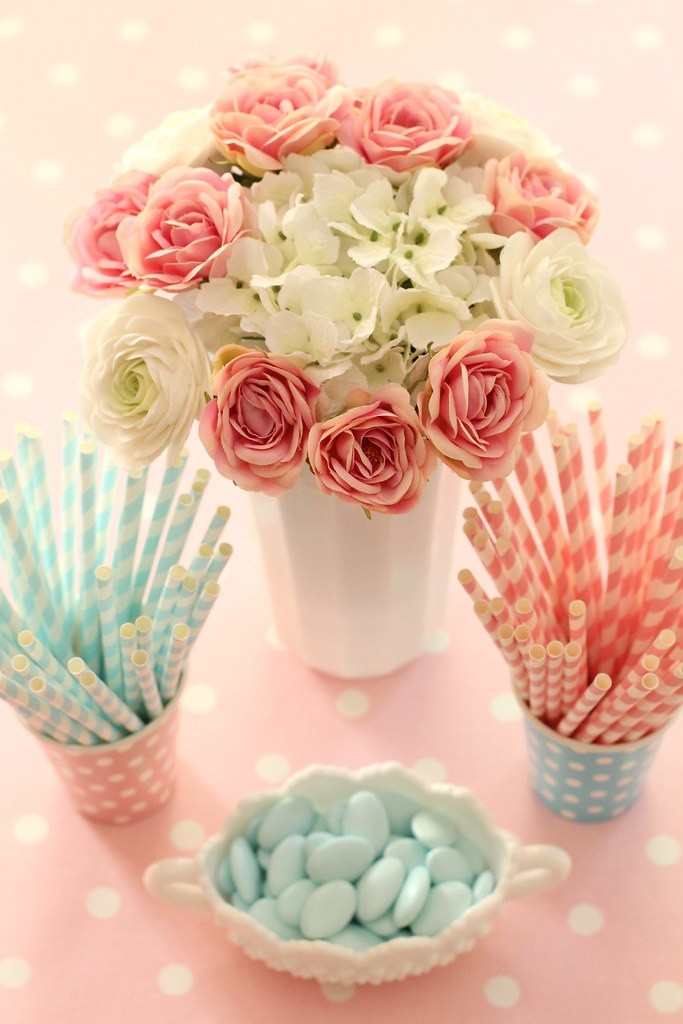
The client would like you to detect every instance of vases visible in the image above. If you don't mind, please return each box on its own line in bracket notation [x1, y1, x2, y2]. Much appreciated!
[248, 448, 463, 679]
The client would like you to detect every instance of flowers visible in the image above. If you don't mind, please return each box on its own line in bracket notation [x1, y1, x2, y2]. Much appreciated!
[49, 52, 631, 520]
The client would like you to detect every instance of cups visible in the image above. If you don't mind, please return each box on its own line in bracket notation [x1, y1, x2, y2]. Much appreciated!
[36, 660, 189, 830]
[524, 703, 669, 821]
